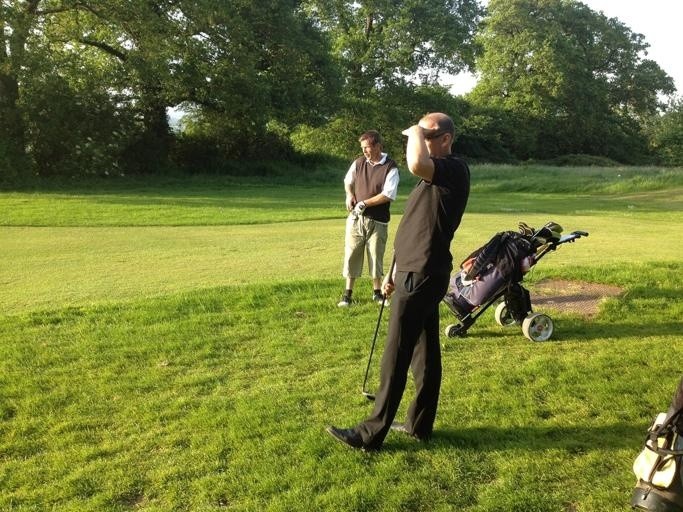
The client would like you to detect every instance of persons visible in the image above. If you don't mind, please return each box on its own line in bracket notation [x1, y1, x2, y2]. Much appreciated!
[325, 112, 469, 452]
[334, 130, 399, 309]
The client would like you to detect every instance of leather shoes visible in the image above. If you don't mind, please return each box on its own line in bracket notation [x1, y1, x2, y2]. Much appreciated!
[389, 420, 431, 443]
[326, 424, 370, 455]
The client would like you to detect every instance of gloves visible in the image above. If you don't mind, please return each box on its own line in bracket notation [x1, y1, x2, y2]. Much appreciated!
[354, 201, 365, 216]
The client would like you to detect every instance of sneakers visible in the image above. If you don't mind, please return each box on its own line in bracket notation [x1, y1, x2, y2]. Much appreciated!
[372, 292, 390, 307]
[337, 294, 351, 308]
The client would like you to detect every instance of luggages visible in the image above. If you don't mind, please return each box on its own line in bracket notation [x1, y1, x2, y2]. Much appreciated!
[628, 379, 683, 511]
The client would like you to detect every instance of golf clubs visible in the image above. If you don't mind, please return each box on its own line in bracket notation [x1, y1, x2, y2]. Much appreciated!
[361, 251, 396, 400]
[516, 222, 564, 246]
